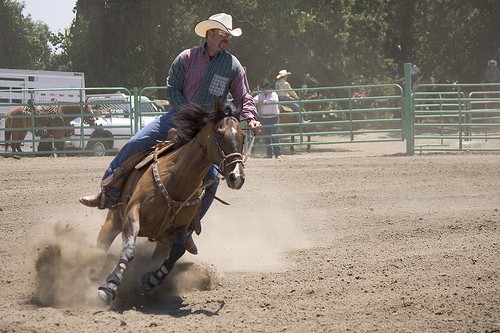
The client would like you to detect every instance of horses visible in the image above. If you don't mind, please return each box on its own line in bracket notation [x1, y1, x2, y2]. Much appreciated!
[5, 104, 98, 152]
[95, 97, 246, 297]
[249, 95, 326, 153]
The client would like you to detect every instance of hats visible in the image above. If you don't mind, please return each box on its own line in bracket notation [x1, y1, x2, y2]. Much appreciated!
[194, 12, 242, 37]
[488, 59, 497, 66]
[276, 69, 291, 79]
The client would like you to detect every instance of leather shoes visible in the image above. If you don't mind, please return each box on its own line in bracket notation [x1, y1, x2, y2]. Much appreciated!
[79, 191, 118, 210]
[184, 233, 198, 254]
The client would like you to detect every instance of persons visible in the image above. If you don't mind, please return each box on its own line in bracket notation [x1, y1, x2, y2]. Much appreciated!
[276, 69, 312, 127]
[394, 59, 500, 109]
[79, 12, 261, 255]
[253, 81, 282, 160]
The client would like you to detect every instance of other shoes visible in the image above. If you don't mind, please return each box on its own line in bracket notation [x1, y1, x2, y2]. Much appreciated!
[276, 155, 281, 159]
[264, 155, 272, 158]
[297, 117, 311, 127]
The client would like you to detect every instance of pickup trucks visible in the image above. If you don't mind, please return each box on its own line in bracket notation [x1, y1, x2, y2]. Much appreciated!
[69, 94, 163, 156]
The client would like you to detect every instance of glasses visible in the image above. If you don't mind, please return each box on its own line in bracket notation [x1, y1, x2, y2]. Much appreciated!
[212, 29, 233, 40]
